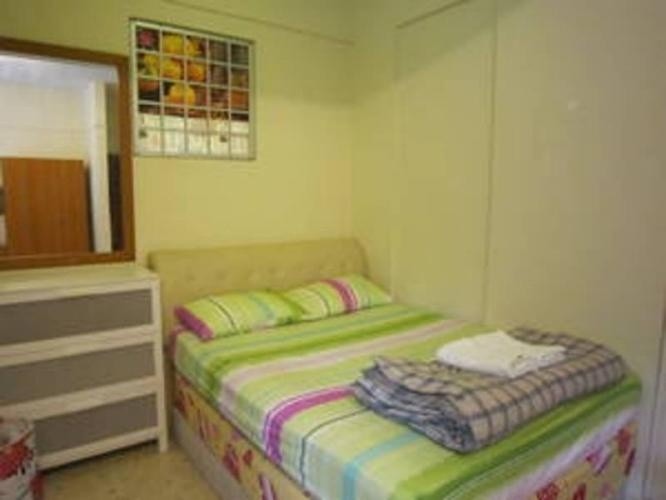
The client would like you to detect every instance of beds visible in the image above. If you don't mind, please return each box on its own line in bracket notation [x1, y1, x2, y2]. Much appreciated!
[146, 232, 642, 500]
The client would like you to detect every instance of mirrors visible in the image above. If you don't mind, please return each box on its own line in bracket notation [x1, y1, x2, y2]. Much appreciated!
[0, 35, 138, 266]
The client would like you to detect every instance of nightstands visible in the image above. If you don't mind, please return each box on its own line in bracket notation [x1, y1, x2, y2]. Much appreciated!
[0, 264, 168, 475]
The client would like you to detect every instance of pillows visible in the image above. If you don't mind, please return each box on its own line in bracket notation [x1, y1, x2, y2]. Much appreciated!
[284, 271, 395, 323]
[175, 290, 295, 336]
